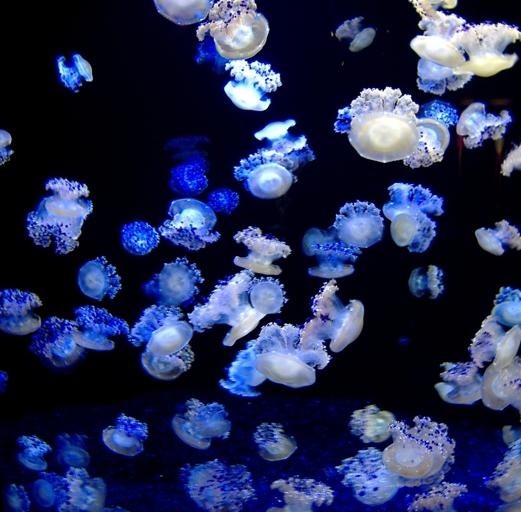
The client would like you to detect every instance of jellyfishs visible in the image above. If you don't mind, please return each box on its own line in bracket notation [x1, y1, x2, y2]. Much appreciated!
[0, 0, 521, 512]
